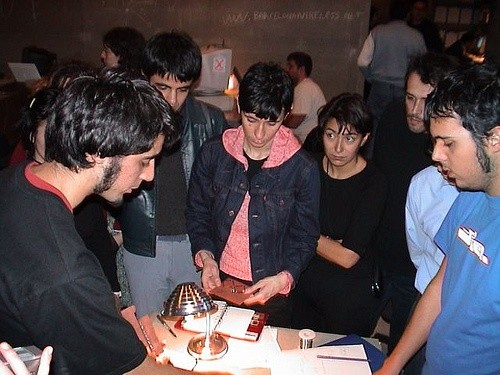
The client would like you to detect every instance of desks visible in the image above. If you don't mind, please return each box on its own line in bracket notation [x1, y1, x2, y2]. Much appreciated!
[138, 303, 383, 375]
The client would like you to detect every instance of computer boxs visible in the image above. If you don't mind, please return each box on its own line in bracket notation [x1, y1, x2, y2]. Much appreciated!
[193, 94, 235, 112]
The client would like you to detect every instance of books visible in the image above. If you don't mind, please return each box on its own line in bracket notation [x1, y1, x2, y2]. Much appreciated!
[174, 300, 270, 342]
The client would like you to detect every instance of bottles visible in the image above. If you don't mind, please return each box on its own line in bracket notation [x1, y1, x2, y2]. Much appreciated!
[299, 328, 316, 349]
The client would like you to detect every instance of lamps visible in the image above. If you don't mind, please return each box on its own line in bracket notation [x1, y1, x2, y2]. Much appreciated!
[160, 281, 228, 361]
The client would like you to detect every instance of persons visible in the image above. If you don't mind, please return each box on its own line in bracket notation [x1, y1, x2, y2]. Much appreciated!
[100, 26, 146, 70]
[407, 0, 442, 50]
[99, 30, 231, 315]
[371, 30, 500, 375]
[289, 92, 386, 331]
[0, 62, 178, 375]
[186, 61, 322, 327]
[358, 0, 425, 115]
[284, 51, 326, 144]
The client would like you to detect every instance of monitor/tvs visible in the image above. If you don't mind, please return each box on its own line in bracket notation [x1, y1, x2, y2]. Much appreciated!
[193, 45, 232, 96]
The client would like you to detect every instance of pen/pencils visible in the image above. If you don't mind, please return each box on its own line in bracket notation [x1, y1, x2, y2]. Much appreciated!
[317, 354, 369, 362]
[156, 314, 177, 338]
